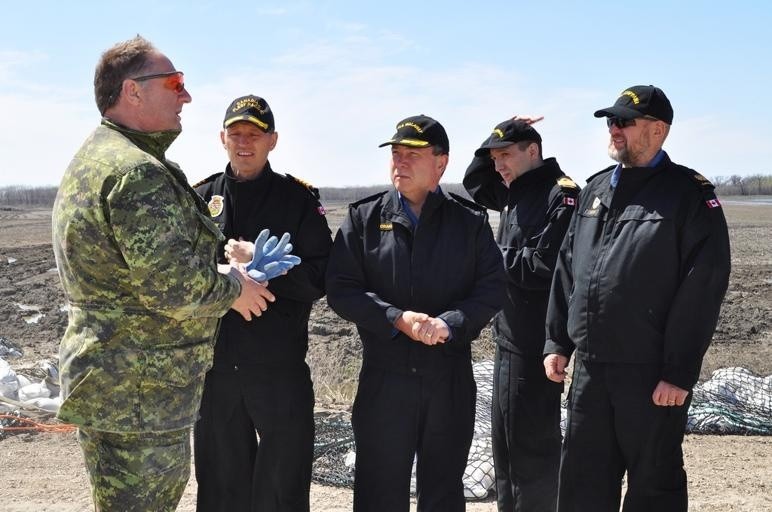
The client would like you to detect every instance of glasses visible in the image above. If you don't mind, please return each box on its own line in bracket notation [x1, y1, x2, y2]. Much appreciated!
[607, 116, 657, 129]
[133, 71, 184, 94]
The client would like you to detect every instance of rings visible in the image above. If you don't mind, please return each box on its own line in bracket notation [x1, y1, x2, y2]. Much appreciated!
[667, 400, 675, 405]
[426, 333, 433, 338]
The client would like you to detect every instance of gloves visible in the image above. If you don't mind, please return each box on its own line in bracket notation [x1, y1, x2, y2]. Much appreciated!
[245, 229, 301, 282]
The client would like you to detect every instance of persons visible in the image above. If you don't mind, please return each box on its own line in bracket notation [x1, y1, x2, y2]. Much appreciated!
[191, 95, 334, 511]
[462, 115, 580, 511]
[51, 37, 277, 512]
[542, 85, 731, 511]
[324, 116, 509, 512]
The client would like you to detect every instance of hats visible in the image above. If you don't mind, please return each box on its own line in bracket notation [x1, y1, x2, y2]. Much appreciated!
[223, 95, 275, 133]
[594, 85, 673, 125]
[474, 119, 541, 156]
[378, 114, 448, 148]
